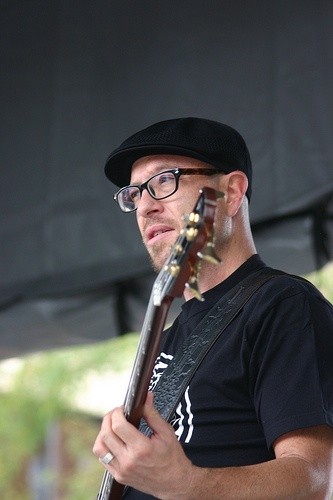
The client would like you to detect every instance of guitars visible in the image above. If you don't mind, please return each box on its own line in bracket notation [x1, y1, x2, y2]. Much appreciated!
[95, 186, 226, 500]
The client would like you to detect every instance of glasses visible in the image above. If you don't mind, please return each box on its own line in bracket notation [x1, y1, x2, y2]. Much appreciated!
[113, 168, 231, 213]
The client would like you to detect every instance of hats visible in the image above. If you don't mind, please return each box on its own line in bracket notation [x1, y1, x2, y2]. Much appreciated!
[105, 118, 252, 204]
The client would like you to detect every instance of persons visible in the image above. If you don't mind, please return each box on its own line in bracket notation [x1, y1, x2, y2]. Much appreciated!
[92, 117, 333, 500]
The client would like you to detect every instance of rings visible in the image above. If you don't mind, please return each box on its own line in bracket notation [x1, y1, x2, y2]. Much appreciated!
[102, 452, 114, 464]
